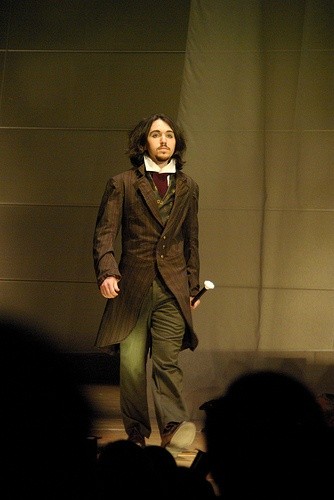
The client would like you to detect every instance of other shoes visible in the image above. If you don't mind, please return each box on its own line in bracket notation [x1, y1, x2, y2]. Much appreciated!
[158, 418, 196, 460]
[125, 433, 147, 449]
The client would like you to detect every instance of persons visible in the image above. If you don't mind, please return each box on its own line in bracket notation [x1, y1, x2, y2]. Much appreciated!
[0, 311, 333, 499]
[90, 111, 203, 453]
